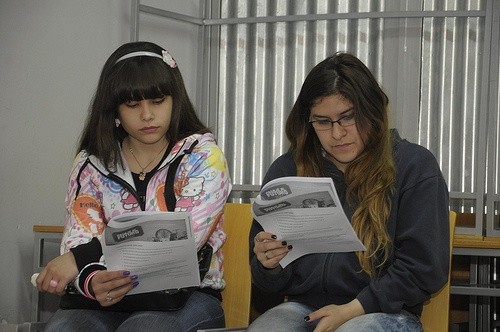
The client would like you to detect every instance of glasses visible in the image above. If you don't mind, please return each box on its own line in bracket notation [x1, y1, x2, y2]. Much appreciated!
[306, 114, 357, 130]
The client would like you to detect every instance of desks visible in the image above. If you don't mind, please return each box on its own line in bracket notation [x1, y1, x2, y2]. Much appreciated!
[31, 224, 67, 321]
[452, 239, 500, 332]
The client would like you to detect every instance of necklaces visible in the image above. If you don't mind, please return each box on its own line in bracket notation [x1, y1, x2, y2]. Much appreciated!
[128, 136, 169, 180]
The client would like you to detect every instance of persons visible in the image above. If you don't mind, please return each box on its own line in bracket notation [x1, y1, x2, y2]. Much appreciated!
[35, 41, 233, 332]
[248, 52, 451, 332]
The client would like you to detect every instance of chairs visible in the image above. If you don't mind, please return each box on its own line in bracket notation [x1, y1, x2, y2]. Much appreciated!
[417, 211, 457, 332]
[220, 202, 254, 327]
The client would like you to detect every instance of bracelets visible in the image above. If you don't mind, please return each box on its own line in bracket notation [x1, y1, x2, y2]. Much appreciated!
[84, 269, 103, 301]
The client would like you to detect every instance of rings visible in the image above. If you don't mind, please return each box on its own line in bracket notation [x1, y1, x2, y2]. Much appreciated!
[105, 290, 114, 302]
[49, 279, 57, 288]
[264, 251, 270, 260]
